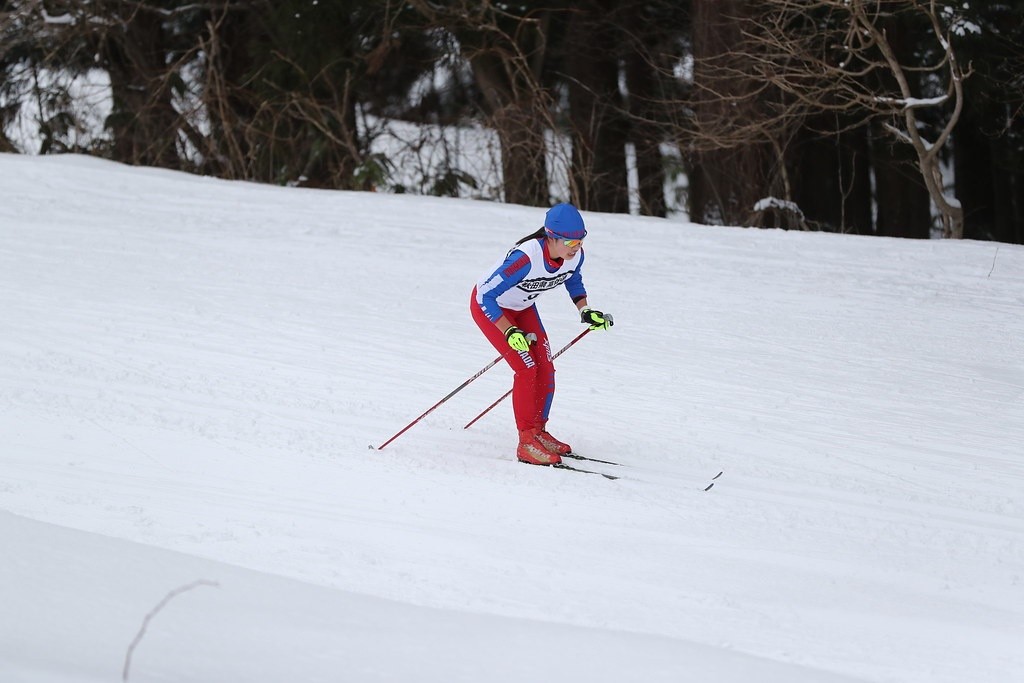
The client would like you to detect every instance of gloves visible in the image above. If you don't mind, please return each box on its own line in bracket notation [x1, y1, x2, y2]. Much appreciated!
[581, 309, 609, 331]
[505, 326, 532, 351]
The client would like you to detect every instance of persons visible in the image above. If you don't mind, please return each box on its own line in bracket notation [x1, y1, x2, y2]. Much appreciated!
[470, 202, 614, 466]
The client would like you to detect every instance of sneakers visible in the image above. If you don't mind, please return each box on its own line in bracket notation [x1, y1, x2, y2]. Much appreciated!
[518, 437, 561, 465]
[534, 430, 571, 454]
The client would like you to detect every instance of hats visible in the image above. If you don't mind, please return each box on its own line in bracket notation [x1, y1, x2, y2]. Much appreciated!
[544, 202, 587, 240]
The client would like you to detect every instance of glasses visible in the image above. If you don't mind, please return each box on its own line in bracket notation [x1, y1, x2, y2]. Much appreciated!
[558, 237, 583, 248]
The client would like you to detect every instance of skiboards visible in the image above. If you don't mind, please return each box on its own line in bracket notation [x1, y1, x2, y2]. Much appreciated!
[500, 449, 724, 492]
